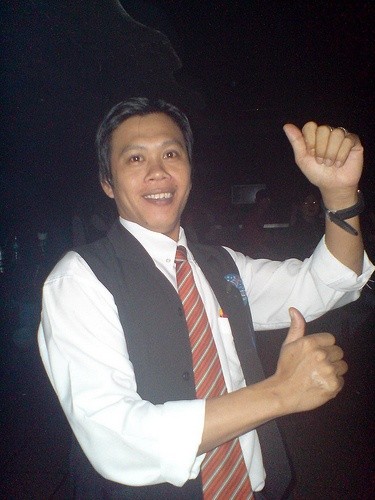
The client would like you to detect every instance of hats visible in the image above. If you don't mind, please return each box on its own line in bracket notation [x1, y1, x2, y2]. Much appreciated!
[256, 190, 271, 199]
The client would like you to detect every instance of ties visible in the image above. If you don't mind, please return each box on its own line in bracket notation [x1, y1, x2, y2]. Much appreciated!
[175, 245, 254, 500]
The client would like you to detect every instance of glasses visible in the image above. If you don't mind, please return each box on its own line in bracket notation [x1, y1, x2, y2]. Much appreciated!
[304, 201, 319, 207]
